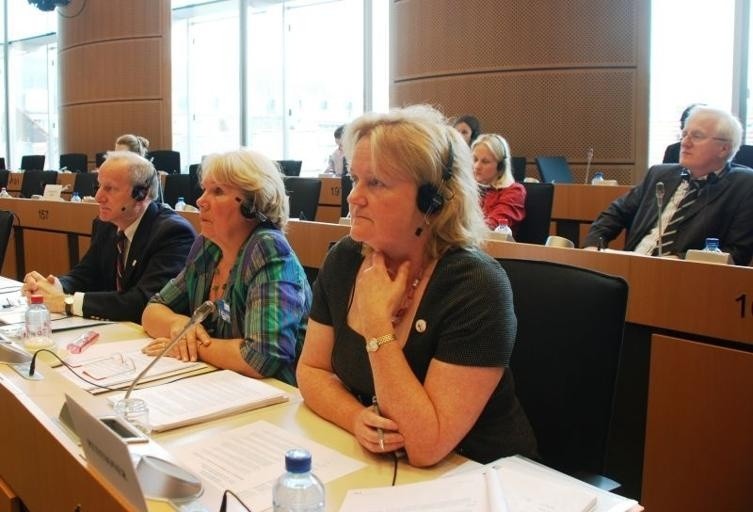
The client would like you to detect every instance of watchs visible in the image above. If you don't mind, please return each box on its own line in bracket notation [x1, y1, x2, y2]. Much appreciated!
[365, 333, 396, 352]
[65, 295, 73, 316]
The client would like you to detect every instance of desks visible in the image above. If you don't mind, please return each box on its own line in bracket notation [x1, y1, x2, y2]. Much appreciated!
[0, 320, 643, 511]
[0, 198, 753, 511]
[0, 173, 632, 252]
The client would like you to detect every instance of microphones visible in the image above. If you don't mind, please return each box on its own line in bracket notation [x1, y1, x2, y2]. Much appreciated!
[235, 196, 255, 215]
[583, 147, 594, 184]
[416, 197, 443, 236]
[122, 299, 216, 421]
[497, 164, 506, 179]
[654, 180, 665, 255]
[122, 189, 145, 212]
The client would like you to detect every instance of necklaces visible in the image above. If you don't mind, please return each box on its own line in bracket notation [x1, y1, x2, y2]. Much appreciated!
[212, 263, 232, 305]
[391, 262, 427, 327]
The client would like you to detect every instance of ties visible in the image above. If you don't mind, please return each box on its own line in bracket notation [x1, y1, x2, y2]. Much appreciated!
[651, 179, 707, 258]
[115, 231, 127, 296]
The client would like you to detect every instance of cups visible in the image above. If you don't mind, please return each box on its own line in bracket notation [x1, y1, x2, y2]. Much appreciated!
[114, 398, 153, 437]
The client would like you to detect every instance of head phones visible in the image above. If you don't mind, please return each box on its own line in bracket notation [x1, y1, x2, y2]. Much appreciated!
[491, 133, 507, 172]
[241, 200, 283, 230]
[132, 167, 157, 201]
[471, 115, 480, 140]
[418, 126, 455, 214]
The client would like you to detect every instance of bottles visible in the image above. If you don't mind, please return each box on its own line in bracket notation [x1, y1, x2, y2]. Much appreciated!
[329, 169, 336, 177]
[0, 187, 8, 198]
[174, 197, 186, 211]
[24, 295, 52, 346]
[701, 238, 721, 252]
[494, 219, 512, 236]
[592, 172, 605, 185]
[272, 449, 326, 512]
[71, 192, 81, 202]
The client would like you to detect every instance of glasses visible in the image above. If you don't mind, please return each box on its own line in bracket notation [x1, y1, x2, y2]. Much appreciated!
[677, 132, 730, 143]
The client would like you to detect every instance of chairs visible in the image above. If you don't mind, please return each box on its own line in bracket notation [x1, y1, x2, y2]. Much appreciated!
[732, 144, 749, 166]
[492, 257, 631, 491]
[510, 156, 526, 182]
[515, 182, 554, 245]
[535, 156, 573, 184]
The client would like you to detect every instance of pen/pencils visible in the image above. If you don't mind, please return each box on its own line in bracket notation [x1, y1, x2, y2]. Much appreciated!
[372, 395, 385, 451]
[598, 236, 602, 250]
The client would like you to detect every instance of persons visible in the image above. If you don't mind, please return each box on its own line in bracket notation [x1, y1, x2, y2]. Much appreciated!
[116, 134, 164, 203]
[662, 104, 709, 163]
[323, 124, 348, 177]
[586, 105, 753, 267]
[296, 102, 543, 469]
[453, 115, 481, 147]
[471, 133, 527, 238]
[22, 151, 197, 326]
[142, 149, 314, 388]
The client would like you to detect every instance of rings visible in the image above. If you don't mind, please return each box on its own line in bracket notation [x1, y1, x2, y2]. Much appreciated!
[162, 343, 165, 348]
